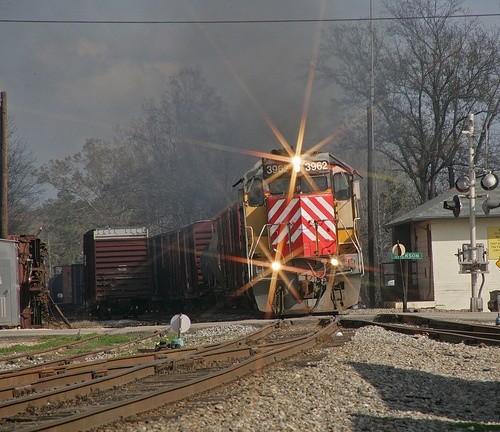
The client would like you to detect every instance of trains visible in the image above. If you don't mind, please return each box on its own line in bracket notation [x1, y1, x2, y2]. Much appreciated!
[49, 143, 367, 317]
